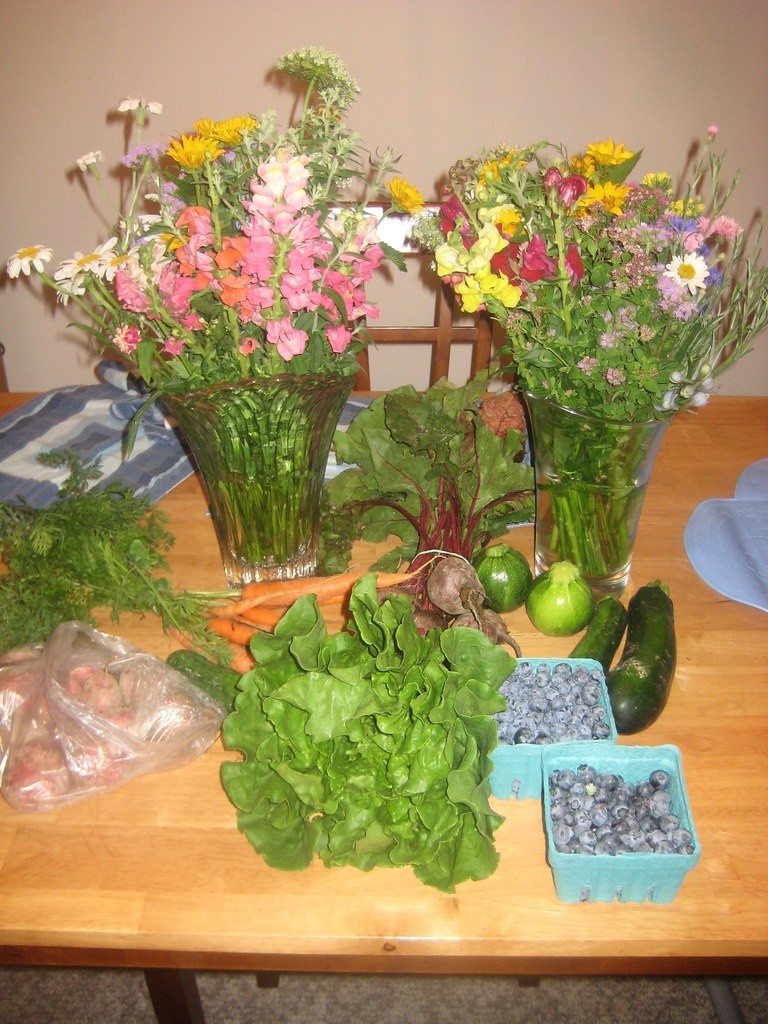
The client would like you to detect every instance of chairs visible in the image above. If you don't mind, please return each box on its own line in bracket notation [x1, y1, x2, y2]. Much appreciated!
[321, 200, 494, 391]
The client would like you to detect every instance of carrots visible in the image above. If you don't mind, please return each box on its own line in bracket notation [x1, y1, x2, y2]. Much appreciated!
[200, 555, 445, 674]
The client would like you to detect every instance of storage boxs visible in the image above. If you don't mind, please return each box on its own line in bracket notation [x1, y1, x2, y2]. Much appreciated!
[541, 743, 703, 903]
[486, 658, 619, 799]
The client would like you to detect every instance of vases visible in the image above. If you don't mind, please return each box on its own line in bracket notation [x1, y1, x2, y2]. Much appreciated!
[156, 374, 358, 586]
[520, 391, 675, 602]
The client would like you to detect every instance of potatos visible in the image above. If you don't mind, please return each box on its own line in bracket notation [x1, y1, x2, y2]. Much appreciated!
[1, 642, 207, 811]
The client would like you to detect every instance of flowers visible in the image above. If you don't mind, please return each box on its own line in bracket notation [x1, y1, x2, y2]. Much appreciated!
[395, 123, 768, 580]
[5, 44, 427, 567]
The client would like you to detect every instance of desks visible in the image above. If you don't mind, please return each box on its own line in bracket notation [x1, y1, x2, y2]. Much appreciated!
[0, 391, 768, 1024]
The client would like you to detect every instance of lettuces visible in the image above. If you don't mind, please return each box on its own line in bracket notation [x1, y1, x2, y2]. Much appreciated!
[219, 573, 508, 896]
